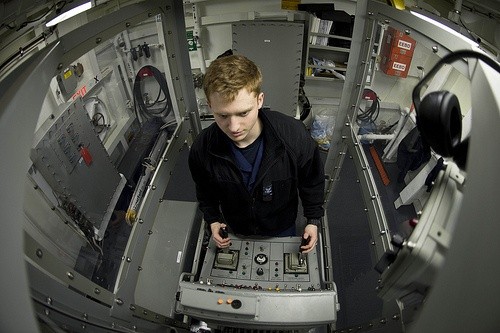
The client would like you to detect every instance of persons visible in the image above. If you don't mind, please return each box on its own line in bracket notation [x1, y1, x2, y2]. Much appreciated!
[188, 55, 324, 255]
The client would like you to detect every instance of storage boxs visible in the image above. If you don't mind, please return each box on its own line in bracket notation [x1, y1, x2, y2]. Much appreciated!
[380, 27, 417, 77]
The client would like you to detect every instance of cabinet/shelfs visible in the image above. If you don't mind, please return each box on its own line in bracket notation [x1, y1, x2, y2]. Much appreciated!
[302, 13, 387, 87]
